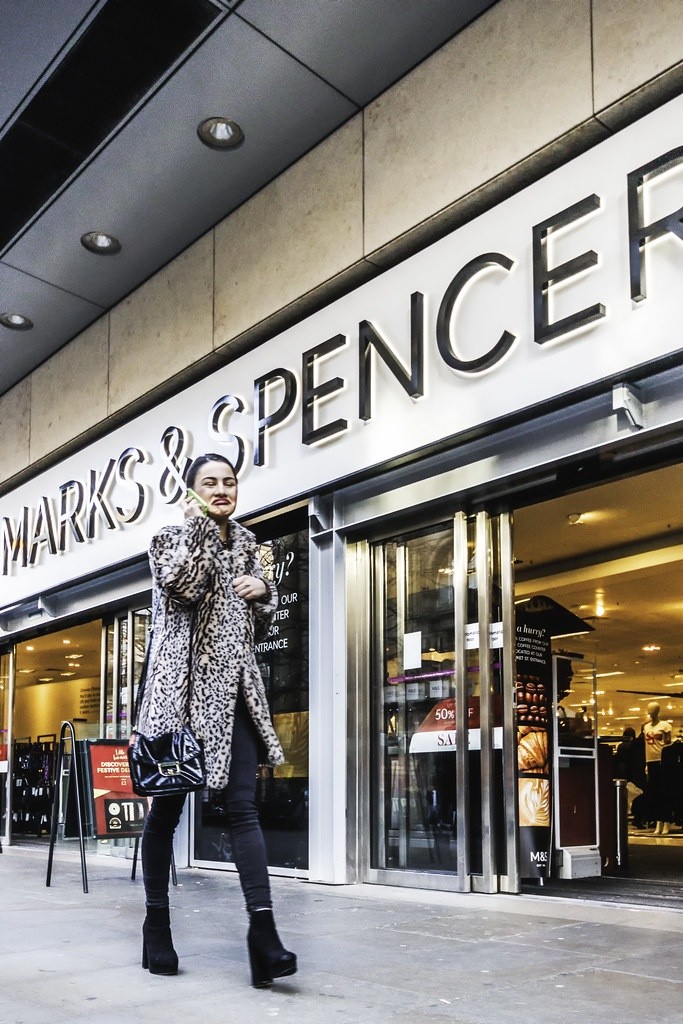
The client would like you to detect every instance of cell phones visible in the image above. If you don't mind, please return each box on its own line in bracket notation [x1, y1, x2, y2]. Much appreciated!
[185, 488, 208, 516]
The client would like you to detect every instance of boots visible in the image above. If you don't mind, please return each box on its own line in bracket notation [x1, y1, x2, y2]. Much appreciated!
[142, 907, 179, 974]
[247, 910, 298, 988]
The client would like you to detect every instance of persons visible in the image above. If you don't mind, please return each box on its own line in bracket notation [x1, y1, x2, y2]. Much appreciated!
[615, 728, 636, 751]
[641, 702, 672, 834]
[133, 453, 299, 987]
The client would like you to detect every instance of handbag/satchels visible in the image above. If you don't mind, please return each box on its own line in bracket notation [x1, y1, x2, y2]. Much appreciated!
[128, 726, 206, 797]
